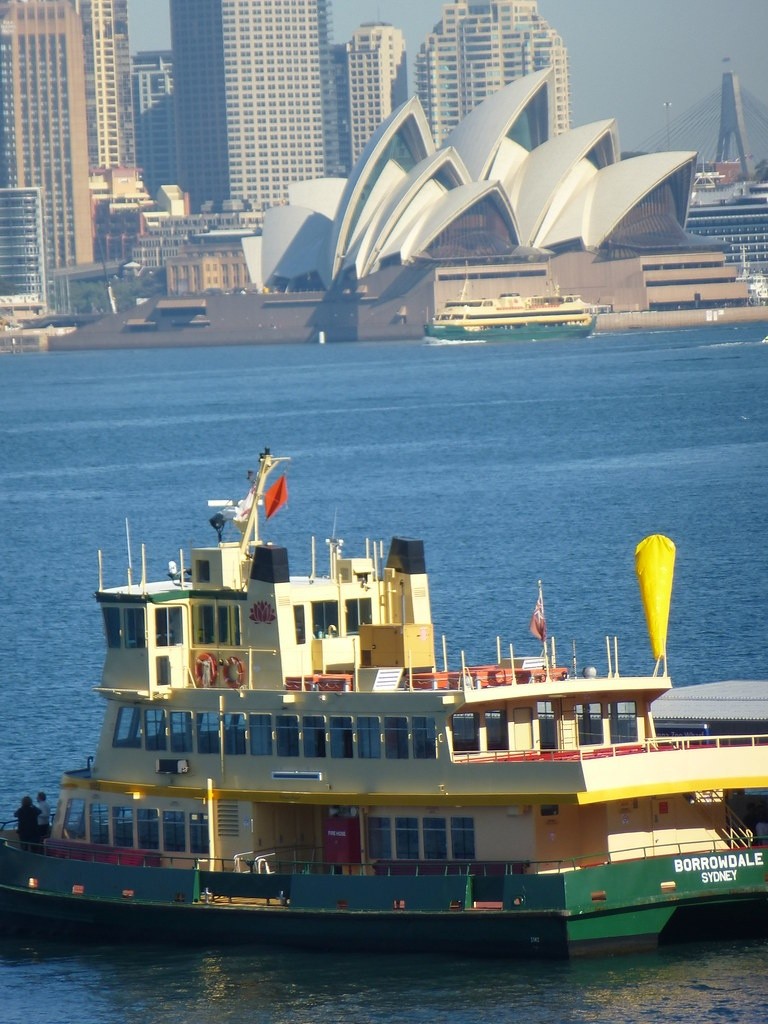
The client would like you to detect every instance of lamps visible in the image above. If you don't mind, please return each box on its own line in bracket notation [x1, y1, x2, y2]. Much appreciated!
[685, 794, 696, 805]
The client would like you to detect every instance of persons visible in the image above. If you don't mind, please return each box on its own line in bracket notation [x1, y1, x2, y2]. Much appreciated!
[13, 792, 50, 852]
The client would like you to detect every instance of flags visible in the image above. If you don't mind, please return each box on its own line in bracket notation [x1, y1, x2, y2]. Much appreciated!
[529, 580, 546, 642]
[264, 471, 287, 520]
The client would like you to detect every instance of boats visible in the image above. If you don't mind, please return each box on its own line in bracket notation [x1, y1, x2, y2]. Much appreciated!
[422, 253, 604, 342]
[0, 443, 767, 965]
